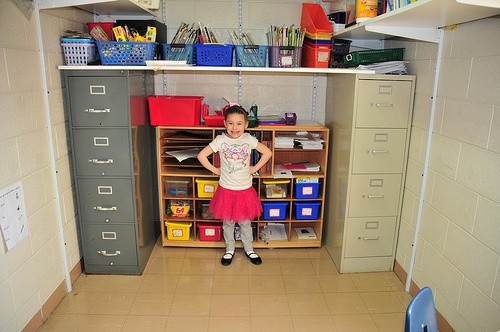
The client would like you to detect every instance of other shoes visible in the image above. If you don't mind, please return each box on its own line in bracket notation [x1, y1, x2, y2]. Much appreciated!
[222, 251, 235, 264]
[245, 251, 262, 265]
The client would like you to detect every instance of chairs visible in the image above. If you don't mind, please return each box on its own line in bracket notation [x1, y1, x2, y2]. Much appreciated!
[404, 286, 438, 332]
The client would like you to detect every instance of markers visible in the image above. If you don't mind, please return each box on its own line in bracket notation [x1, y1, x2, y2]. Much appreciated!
[259, 237, 269, 244]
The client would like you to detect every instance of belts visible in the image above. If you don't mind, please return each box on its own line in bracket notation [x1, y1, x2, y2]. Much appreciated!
[344, 47, 405, 65]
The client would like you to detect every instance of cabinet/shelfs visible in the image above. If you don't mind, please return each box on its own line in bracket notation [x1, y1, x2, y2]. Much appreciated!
[325, 75, 418, 275]
[59, 65, 154, 275]
[156, 120, 330, 249]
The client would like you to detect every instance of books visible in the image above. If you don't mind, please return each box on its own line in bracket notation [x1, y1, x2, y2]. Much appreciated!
[263, 222, 287, 240]
[64, 20, 306, 67]
[295, 227, 316, 239]
[274, 132, 325, 178]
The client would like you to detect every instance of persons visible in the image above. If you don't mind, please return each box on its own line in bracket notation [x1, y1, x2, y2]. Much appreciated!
[197, 105, 272, 265]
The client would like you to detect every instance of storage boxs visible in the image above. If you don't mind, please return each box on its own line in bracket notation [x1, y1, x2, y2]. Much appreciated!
[147, 95, 204, 127]
[301, 3, 334, 68]
[163, 178, 320, 241]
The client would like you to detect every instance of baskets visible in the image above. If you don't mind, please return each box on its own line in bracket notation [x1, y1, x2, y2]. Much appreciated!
[269, 46, 301, 68]
[235, 45, 268, 67]
[162, 43, 194, 64]
[60, 38, 99, 66]
[97, 40, 158, 65]
[195, 44, 234, 67]
